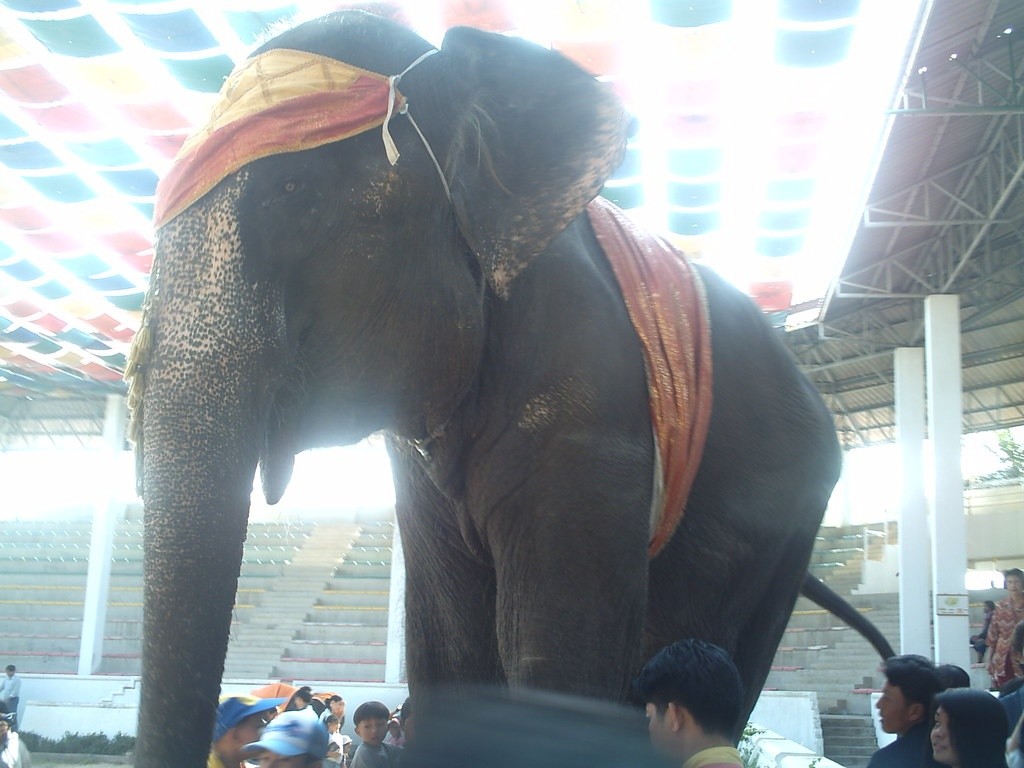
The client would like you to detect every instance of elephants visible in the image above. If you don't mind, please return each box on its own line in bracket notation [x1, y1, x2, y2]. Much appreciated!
[128, 6, 896, 768]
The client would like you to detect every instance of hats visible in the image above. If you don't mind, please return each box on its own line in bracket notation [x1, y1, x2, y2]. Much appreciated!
[343, 735, 352, 745]
[241, 710, 329, 760]
[212, 694, 288, 743]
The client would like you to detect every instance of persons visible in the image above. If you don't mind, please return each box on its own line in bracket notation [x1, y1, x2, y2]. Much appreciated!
[638, 641, 745, 768]
[999, 619, 1024, 768]
[937, 664, 970, 689]
[0, 719, 30, 768]
[319, 695, 345, 725]
[348, 701, 403, 768]
[867, 654, 944, 768]
[924, 688, 1008, 768]
[207, 696, 288, 768]
[985, 568, 1024, 689]
[341, 735, 352, 768]
[384, 718, 405, 745]
[323, 715, 343, 768]
[240, 711, 329, 768]
[393, 697, 414, 768]
[0, 665, 19, 732]
[971, 600, 994, 640]
[285, 686, 312, 711]
